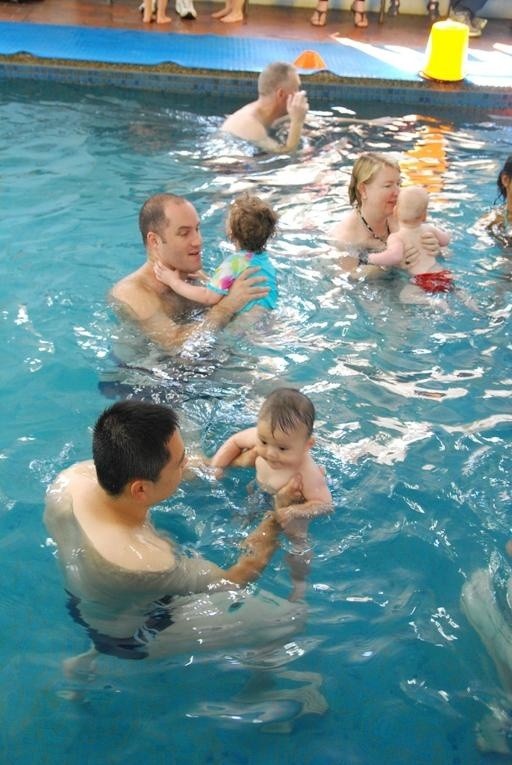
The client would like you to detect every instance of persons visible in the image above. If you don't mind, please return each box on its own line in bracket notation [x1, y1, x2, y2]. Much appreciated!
[218, 61, 404, 156]
[137, 0, 246, 24]
[209, 387, 334, 604]
[388, 1, 488, 36]
[473, 154, 511, 313]
[327, 150, 452, 350]
[41, 399, 326, 711]
[96, 191, 271, 482]
[311, 0, 370, 27]
[345, 186, 481, 315]
[153, 194, 280, 341]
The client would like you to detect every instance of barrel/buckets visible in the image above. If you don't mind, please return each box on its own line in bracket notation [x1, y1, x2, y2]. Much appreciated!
[418, 20, 471, 84]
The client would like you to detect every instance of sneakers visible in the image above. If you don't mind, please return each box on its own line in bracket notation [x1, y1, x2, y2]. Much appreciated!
[447, 11, 482, 36]
[174, 0, 197, 20]
[138, 0, 159, 13]
[471, 15, 489, 29]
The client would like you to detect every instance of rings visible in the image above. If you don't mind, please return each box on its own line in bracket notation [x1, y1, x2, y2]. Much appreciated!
[405, 258, 410, 264]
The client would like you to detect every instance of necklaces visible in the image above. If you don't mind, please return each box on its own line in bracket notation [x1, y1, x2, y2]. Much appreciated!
[356, 207, 391, 244]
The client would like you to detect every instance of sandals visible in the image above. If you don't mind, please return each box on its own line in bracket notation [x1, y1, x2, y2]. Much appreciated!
[311, 0, 328, 28]
[388, 1, 399, 18]
[427, 1, 439, 23]
[350, 0, 369, 28]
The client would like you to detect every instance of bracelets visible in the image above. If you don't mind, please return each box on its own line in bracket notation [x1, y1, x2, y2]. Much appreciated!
[213, 305, 234, 317]
[358, 252, 367, 266]
